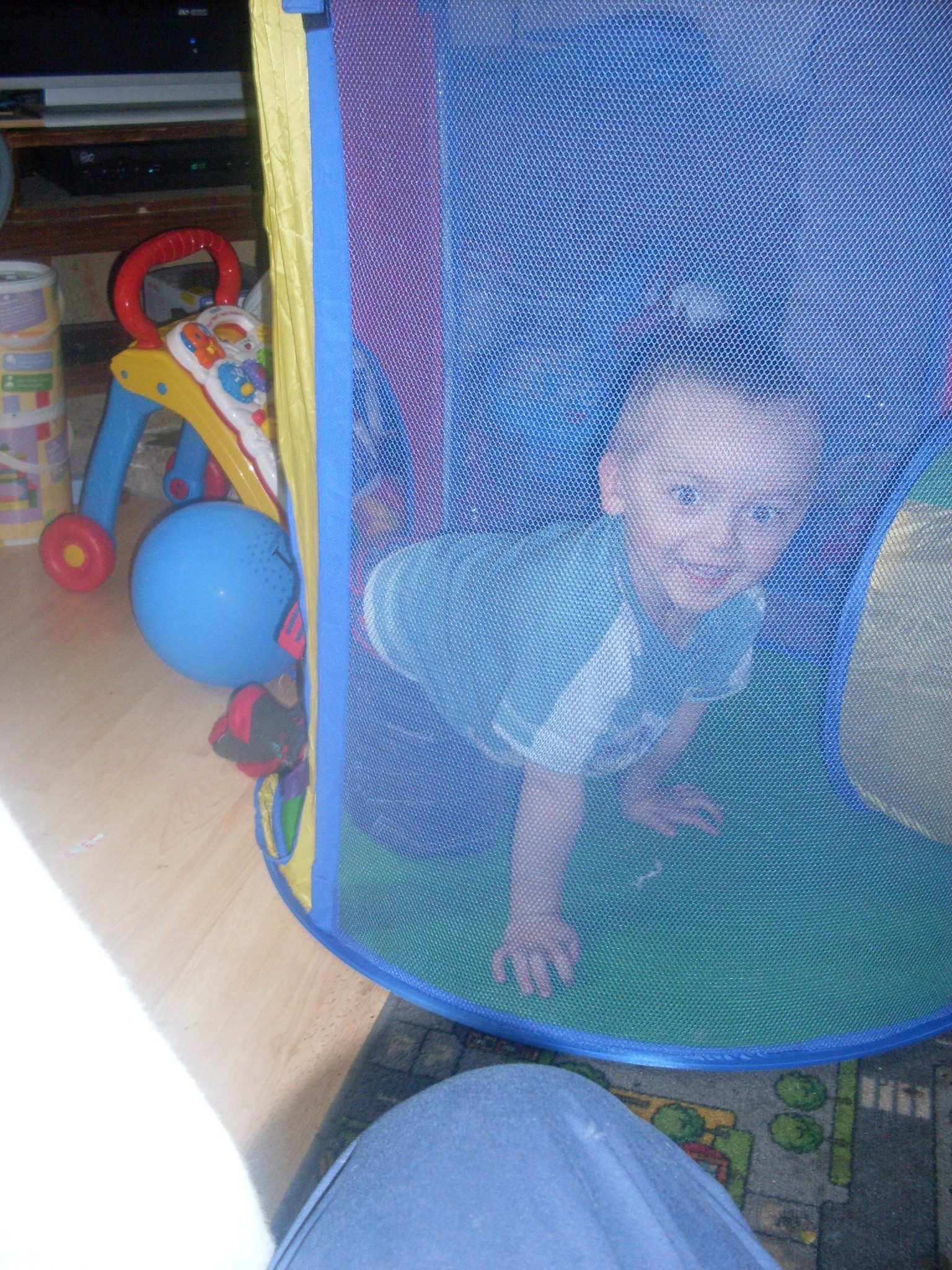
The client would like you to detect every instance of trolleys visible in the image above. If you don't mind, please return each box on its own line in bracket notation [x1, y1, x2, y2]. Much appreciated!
[41, 230, 291, 597]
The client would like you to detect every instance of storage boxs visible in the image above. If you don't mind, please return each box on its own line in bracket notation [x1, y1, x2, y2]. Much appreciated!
[142, 257, 263, 326]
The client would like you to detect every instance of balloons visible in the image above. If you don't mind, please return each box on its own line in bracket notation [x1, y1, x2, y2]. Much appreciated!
[130, 500, 308, 694]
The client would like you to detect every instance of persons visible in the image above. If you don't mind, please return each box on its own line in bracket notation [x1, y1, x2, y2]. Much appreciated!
[266, 1062, 782, 1270]
[345, 348, 822, 1000]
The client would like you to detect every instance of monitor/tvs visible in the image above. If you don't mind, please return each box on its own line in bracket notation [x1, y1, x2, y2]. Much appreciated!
[0, 0, 254, 107]
